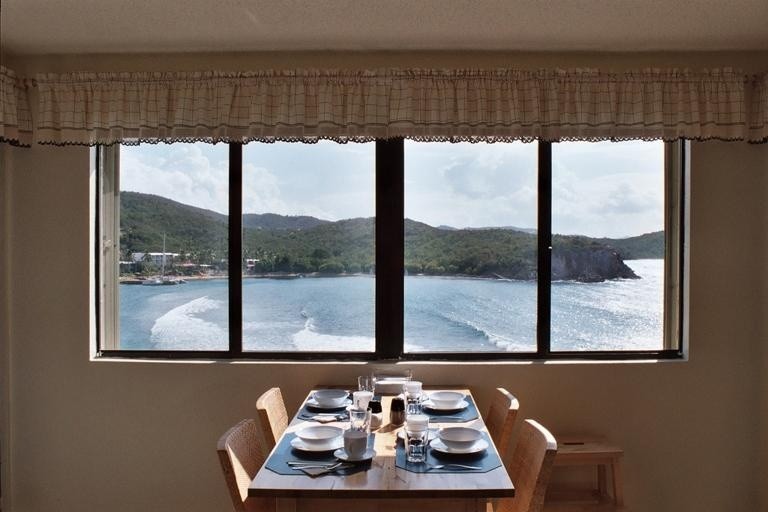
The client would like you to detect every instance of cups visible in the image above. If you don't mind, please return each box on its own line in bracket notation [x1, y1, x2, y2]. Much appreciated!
[353, 391, 372, 408]
[403, 381, 421, 414]
[404, 414, 429, 463]
[342, 430, 368, 455]
[349, 407, 372, 436]
[356, 375, 376, 391]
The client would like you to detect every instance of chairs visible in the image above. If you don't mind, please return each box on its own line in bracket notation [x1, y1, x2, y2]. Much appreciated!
[217, 419, 276, 512]
[486, 387, 519, 462]
[255, 388, 288, 451]
[486, 418, 557, 512]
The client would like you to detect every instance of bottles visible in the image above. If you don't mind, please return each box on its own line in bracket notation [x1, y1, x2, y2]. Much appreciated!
[367, 400, 384, 428]
[389, 398, 405, 427]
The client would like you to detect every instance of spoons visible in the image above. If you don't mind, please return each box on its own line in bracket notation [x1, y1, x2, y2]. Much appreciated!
[286, 461, 342, 470]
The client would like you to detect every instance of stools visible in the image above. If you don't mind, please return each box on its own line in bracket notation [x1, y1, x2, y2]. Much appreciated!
[544, 435, 624, 512]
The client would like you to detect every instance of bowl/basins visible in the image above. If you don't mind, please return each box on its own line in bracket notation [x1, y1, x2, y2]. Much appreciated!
[312, 389, 350, 405]
[295, 426, 345, 443]
[428, 391, 466, 406]
[434, 427, 487, 449]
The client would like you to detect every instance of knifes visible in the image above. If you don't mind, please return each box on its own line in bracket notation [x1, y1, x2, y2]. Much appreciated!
[303, 410, 362, 418]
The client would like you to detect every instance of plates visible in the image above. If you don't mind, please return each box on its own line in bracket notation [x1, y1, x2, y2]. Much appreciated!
[423, 400, 470, 412]
[429, 438, 489, 455]
[397, 429, 436, 441]
[346, 404, 365, 414]
[334, 447, 377, 461]
[303, 397, 354, 410]
[289, 437, 346, 452]
[397, 391, 429, 402]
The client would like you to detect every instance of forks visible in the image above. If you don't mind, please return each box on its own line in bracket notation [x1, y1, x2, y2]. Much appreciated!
[422, 458, 483, 472]
